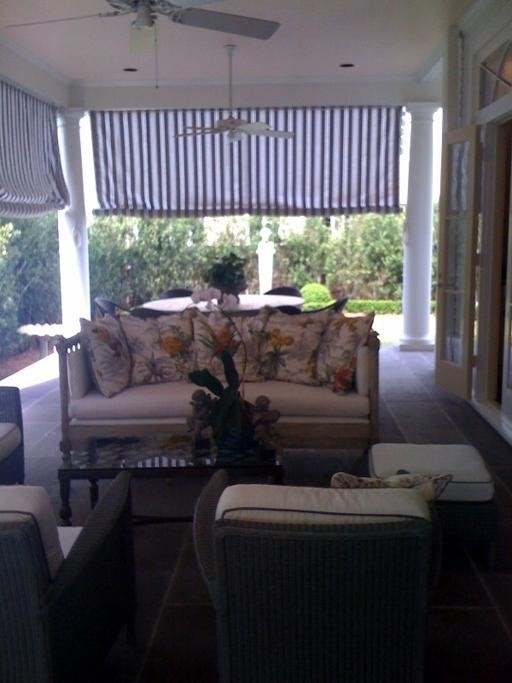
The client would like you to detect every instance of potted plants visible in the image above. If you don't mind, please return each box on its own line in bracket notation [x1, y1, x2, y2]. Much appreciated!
[204, 253, 248, 309]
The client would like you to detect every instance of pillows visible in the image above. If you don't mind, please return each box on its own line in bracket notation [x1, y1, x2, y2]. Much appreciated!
[74, 310, 371, 396]
[326, 467, 453, 506]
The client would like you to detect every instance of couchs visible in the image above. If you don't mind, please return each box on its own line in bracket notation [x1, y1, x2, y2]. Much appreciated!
[48, 308, 385, 524]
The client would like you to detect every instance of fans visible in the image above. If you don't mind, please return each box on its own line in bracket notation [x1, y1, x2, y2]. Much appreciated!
[176, 42, 296, 144]
[0, 0, 289, 62]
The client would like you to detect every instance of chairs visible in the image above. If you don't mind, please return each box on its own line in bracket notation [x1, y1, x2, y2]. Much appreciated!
[1, 471, 139, 682]
[93, 282, 354, 320]
[1, 385, 36, 484]
[186, 465, 443, 683]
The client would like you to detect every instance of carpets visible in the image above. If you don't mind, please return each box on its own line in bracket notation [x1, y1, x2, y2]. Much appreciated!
[131, 537, 512, 683]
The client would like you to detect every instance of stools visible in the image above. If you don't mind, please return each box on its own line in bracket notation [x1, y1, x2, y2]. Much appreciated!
[367, 440, 498, 574]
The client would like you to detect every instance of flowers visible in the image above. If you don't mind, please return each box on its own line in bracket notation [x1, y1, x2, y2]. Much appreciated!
[182, 281, 249, 399]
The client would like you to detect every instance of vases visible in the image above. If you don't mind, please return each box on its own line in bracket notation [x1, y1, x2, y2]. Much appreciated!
[210, 390, 259, 460]
